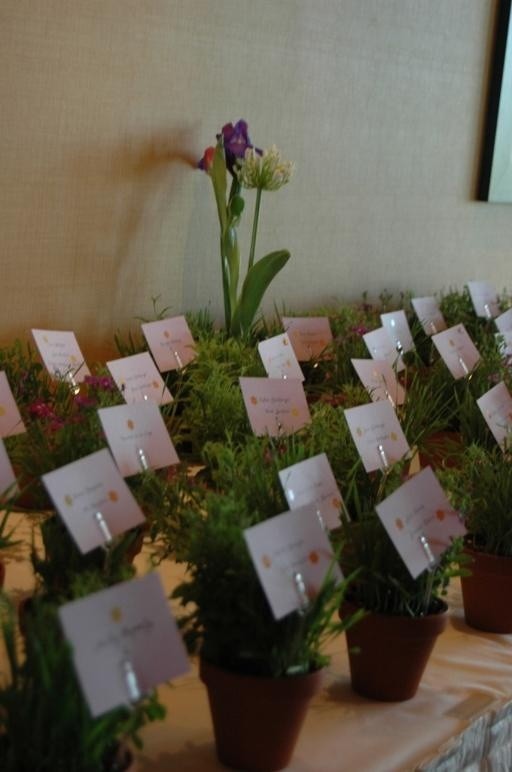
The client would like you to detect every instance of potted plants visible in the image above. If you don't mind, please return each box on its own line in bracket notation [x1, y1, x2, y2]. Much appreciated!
[331, 454, 478, 703]
[454, 437, 512, 634]
[170, 495, 366, 771]
[412, 344, 493, 469]
[40, 402, 175, 567]
[14, 436, 137, 662]
[0, 564, 194, 770]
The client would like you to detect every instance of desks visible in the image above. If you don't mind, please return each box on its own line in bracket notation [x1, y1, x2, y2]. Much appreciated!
[1, 462, 511, 772]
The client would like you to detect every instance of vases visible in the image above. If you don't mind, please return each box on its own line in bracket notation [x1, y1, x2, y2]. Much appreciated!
[12, 457, 49, 513]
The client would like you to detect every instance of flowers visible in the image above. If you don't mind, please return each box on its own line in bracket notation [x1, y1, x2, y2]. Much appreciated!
[194, 117, 299, 338]
[9, 374, 117, 460]
